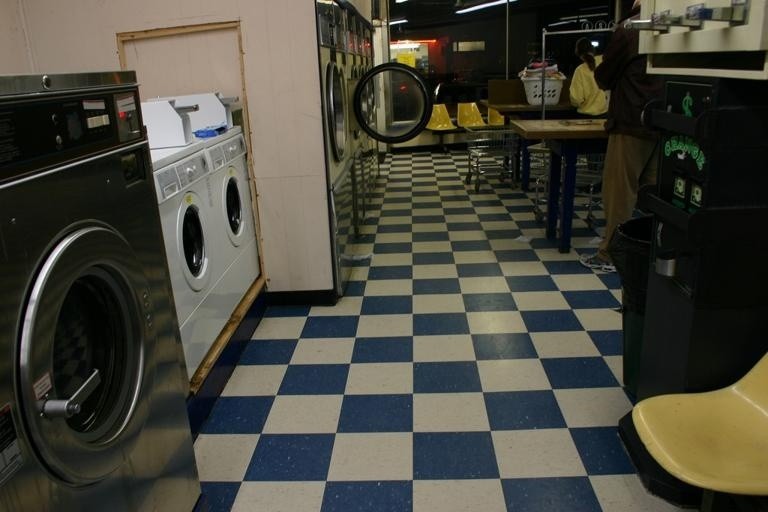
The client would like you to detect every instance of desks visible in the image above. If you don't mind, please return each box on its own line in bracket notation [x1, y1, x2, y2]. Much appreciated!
[478, 98, 608, 253]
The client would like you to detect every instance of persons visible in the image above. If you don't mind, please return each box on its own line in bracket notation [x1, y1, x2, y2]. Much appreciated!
[560, 37, 611, 119]
[579, 9, 663, 273]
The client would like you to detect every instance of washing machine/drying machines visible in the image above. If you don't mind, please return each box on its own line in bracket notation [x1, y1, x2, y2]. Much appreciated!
[315, 0, 435, 298]
[4, 71, 207, 511]
[133, 92, 264, 382]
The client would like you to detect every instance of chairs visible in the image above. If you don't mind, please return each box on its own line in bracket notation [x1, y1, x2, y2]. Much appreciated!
[617, 349, 768, 512]
[425, 102, 487, 152]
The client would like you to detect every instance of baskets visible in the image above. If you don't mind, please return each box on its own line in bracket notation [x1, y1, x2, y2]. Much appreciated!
[521, 71, 567, 106]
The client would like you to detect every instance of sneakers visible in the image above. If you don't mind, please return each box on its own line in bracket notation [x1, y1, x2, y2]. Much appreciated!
[579, 251, 617, 272]
